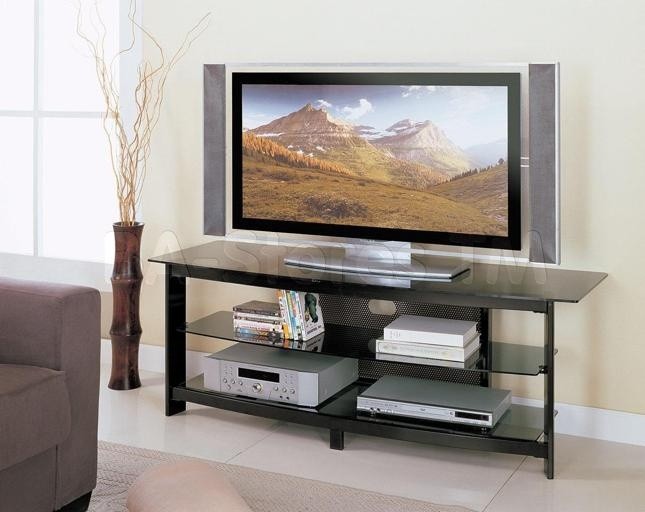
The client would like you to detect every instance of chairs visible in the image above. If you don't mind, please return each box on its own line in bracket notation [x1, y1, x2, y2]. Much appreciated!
[2, 281, 100, 510]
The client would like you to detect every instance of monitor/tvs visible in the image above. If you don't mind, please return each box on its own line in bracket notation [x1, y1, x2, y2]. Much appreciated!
[205, 62, 561, 280]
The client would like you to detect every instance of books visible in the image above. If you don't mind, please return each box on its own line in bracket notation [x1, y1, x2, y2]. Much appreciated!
[375, 314, 482, 363]
[234, 333, 325, 352]
[232, 289, 325, 342]
[376, 351, 480, 370]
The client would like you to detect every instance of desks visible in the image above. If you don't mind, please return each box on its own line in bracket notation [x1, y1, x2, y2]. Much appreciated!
[147, 239, 608, 481]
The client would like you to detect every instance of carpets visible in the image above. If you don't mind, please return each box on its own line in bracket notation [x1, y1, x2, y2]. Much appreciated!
[89, 441, 480, 512]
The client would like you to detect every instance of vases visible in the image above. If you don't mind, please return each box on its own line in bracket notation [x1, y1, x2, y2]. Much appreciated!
[109, 221, 144, 392]
